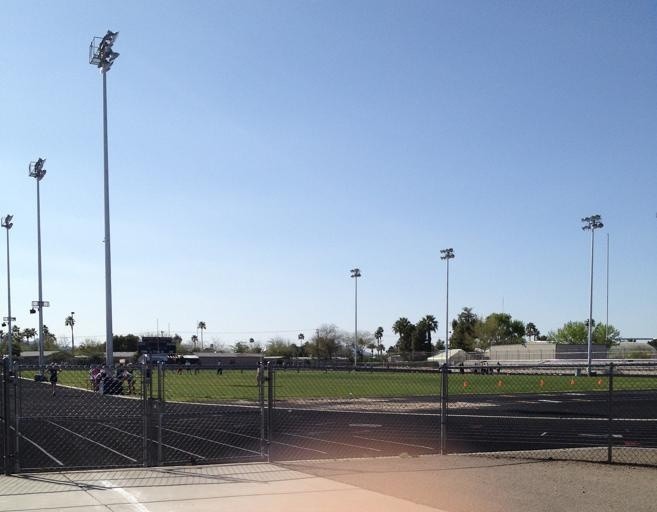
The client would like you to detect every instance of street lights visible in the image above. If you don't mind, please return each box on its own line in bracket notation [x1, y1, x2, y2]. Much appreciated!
[349, 268, 361, 366]
[28, 157, 48, 382]
[88, 30, 123, 379]
[1, 214, 14, 372]
[437, 247, 456, 364]
[64, 311, 76, 357]
[298, 333, 305, 347]
[190, 335, 198, 351]
[580, 213, 603, 377]
[197, 321, 206, 350]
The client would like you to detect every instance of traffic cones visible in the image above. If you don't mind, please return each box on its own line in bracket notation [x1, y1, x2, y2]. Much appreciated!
[496, 378, 503, 388]
[596, 377, 603, 385]
[462, 380, 469, 388]
[538, 377, 544, 387]
[570, 376, 576, 384]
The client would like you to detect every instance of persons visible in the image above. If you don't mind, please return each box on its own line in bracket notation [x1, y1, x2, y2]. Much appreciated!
[43, 357, 264, 397]
[458, 360, 502, 376]
